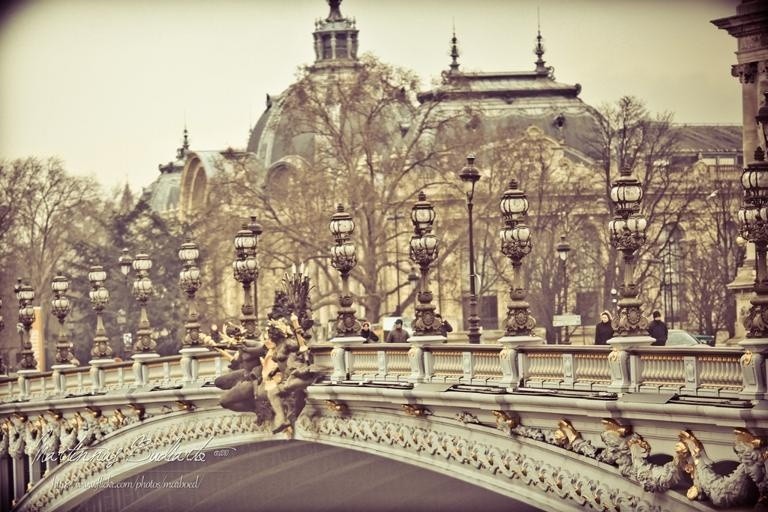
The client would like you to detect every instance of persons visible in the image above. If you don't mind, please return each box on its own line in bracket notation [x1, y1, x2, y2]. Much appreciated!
[595, 311, 613, 345]
[214, 325, 267, 426]
[267, 324, 312, 384]
[386, 319, 410, 343]
[647, 311, 668, 346]
[361, 323, 379, 343]
[434, 314, 453, 344]
